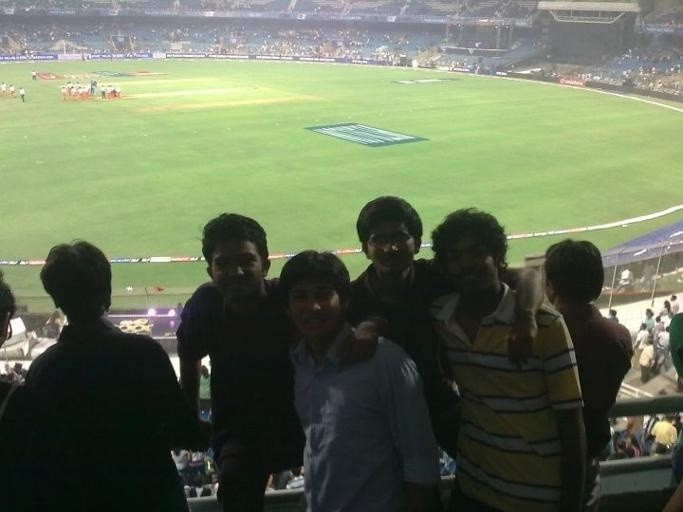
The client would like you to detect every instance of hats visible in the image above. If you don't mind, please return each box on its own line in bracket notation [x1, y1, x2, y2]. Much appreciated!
[187, 451, 205, 467]
[650, 419, 677, 446]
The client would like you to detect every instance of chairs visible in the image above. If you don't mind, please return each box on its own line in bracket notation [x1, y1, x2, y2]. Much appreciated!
[0, 1, 683, 101]
